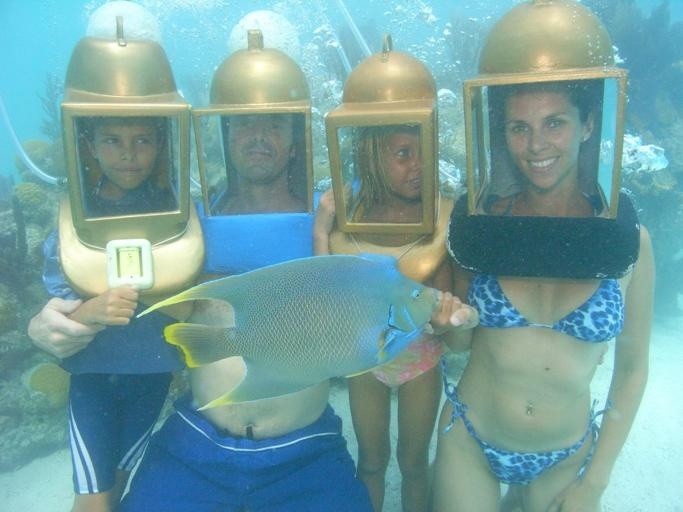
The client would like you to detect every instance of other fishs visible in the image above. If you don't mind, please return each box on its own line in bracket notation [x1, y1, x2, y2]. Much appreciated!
[136, 255, 440, 411]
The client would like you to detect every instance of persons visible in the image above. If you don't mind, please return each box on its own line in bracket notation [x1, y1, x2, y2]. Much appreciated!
[42, 117, 184, 511]
[312, 123, 460, 511]
[430, 76, 655, 511]
[27, 116, 374, 511]
